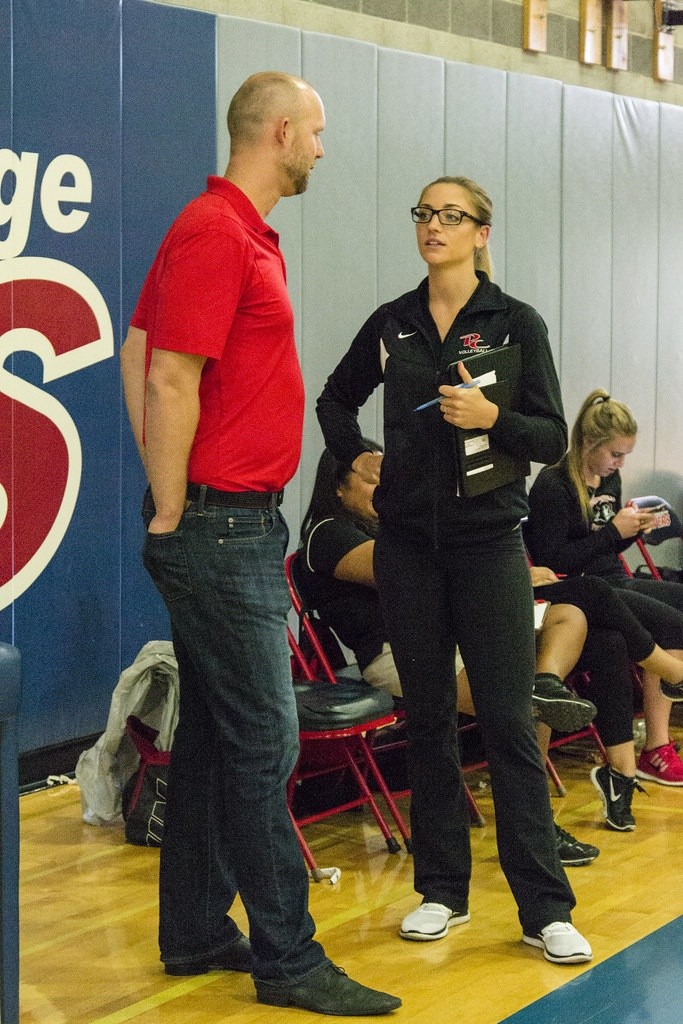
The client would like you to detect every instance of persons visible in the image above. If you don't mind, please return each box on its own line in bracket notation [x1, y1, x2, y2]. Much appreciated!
[521, 387, 682, 832]
[120, 71, 404, 1017]
[297, 438, 601, 867]
[317, 176, 595, 963]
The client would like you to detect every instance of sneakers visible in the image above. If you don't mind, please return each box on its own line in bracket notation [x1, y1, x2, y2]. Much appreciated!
[530, 678, 597, 733]
[635, 740, 682, 787]
[589, 761, 649, 832]
[658, 677, 683, 702]
[399, 895, 471, 942]
[522, 922, 593, 965]
[553, 821, 600, 866]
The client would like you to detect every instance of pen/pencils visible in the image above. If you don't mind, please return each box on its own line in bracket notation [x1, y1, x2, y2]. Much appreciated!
[413, 379, 482, 412]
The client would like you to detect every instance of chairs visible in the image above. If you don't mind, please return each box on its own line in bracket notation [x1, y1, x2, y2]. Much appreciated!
[286, 546, 495, 851]
[538, 715, 613, 795]
[288, 631, 401, 886]
[526, 494, 683, 797]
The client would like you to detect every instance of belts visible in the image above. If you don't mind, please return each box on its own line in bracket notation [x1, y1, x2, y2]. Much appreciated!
[184, 481, 285, 509]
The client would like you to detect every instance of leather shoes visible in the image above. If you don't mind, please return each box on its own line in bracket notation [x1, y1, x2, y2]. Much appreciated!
[166, 934, 251, 977]
[255, 965, 402, 1016]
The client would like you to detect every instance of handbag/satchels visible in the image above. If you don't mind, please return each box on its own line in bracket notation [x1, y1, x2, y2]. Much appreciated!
[122, 715, 171, 847]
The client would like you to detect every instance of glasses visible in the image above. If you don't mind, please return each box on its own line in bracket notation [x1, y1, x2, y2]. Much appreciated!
[410, 207, 489, 226]
